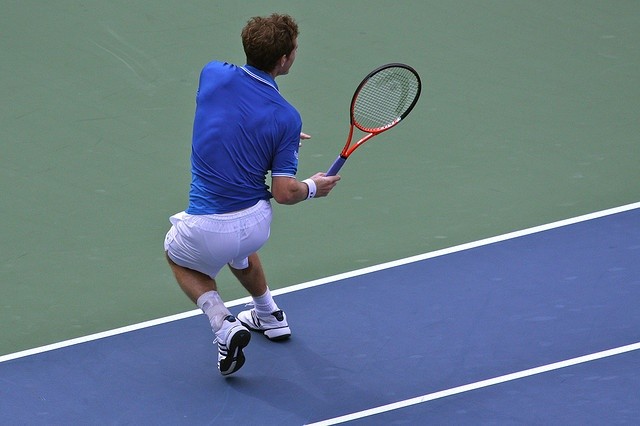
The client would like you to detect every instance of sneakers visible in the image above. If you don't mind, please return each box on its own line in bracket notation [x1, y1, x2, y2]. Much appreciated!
[236, 308, 292, 341]
[213, 314, 252, 376]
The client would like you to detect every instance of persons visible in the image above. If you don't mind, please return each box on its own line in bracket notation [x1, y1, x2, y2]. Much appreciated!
[162, 13, 341, 376]
